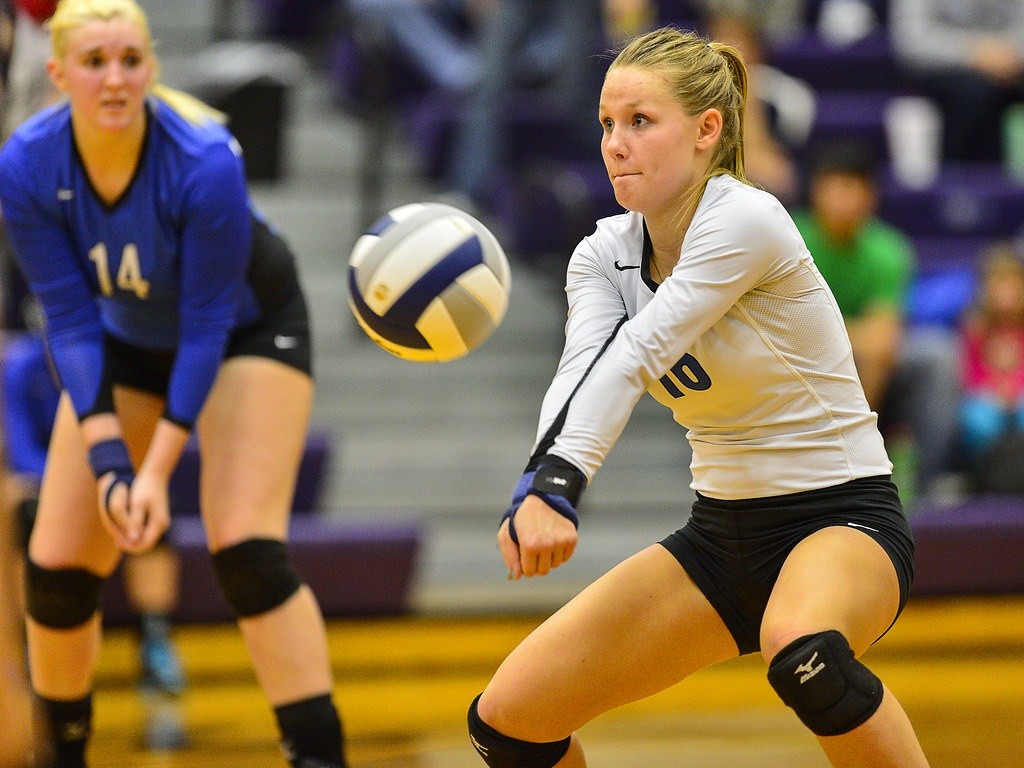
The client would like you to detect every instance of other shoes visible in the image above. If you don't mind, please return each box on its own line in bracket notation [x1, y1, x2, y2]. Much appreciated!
[143, 647, 181, 694]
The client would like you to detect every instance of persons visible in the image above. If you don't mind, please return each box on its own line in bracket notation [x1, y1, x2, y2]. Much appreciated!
[336, 4, 1023, 512]
[465, 24, 931, 768]
[0, 1, 349, 767]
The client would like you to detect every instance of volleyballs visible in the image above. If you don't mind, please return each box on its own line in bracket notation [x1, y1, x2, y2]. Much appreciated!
[342, 199, 515, 363]
